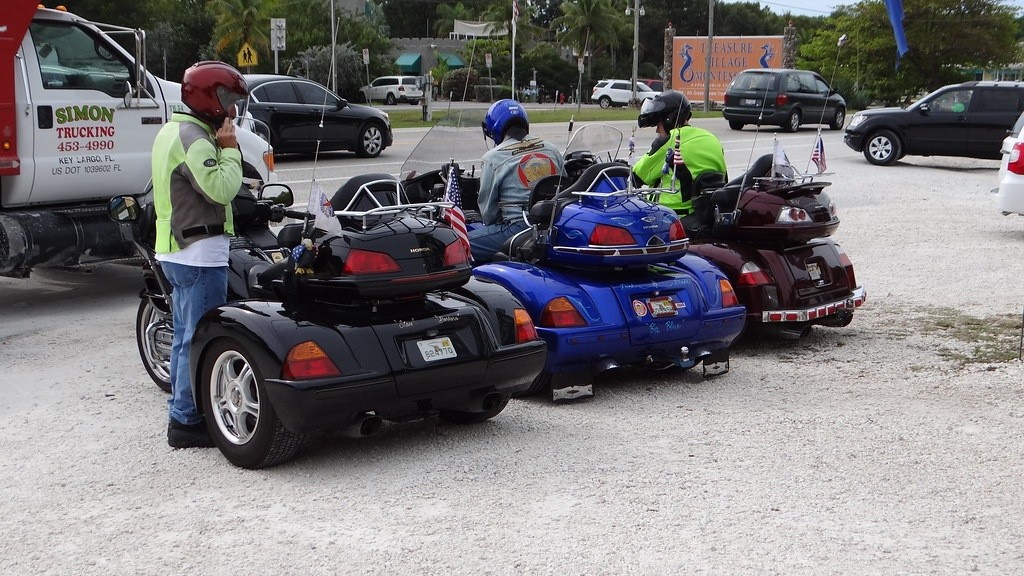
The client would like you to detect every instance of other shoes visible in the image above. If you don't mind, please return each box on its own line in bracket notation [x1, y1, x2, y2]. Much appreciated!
[167, 420, 217, 448]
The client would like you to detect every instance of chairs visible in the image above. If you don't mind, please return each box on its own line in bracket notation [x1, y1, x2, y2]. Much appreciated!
[256, 220, 315, 284]
[503, 173, 569, 252]
[681, 170, 723, 225]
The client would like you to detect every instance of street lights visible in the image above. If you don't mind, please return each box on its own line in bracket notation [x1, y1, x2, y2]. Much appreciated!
[625, 5, 646, 97]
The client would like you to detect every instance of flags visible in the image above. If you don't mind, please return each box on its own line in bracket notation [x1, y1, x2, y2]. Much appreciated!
[773, 139, 794, 179]
[812, 136, 827, 174]
[313, 184, 343, 238]
[444, 167, 470, 260]
[674, 135, 683, 164]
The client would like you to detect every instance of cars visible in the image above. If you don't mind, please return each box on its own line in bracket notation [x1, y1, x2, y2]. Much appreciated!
[722, 68, 846, 134]
[237, 74, 394, 159]
[592, 79, 664, 109]
[638, 79, 664, 91]
[359, 76, 424, 105]
[989, 111, 1024, 217]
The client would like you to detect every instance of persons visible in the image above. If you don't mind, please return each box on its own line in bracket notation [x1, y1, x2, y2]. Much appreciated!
[632, 90, 728, 218]
[464, 99, 568, 262]
[516, 84, 565, 104]
[153, 60, 251, 447]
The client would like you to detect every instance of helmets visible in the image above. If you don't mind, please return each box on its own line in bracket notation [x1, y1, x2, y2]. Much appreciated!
[484, 98, 529, 146]
[181, 61, 250, 123]
[638, 90, 692, 136]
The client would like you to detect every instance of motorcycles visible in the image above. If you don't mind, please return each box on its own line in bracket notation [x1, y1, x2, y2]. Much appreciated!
[399, 117, 747, 402]
[561, 124, 867, 341]
[107, 111, 549, 476]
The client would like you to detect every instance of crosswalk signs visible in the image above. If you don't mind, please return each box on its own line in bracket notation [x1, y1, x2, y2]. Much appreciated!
[236, 42, 258, 66]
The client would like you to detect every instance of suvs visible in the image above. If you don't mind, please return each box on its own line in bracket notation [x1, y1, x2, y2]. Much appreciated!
[844, 78, 1024, 166]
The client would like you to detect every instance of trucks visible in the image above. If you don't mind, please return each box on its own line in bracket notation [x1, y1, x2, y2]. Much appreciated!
[0, 0, 297, 280]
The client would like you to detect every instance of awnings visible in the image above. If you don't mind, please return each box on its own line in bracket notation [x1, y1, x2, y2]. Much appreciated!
[394, 54, 422, 72]
[439, 53, 465, 71]
